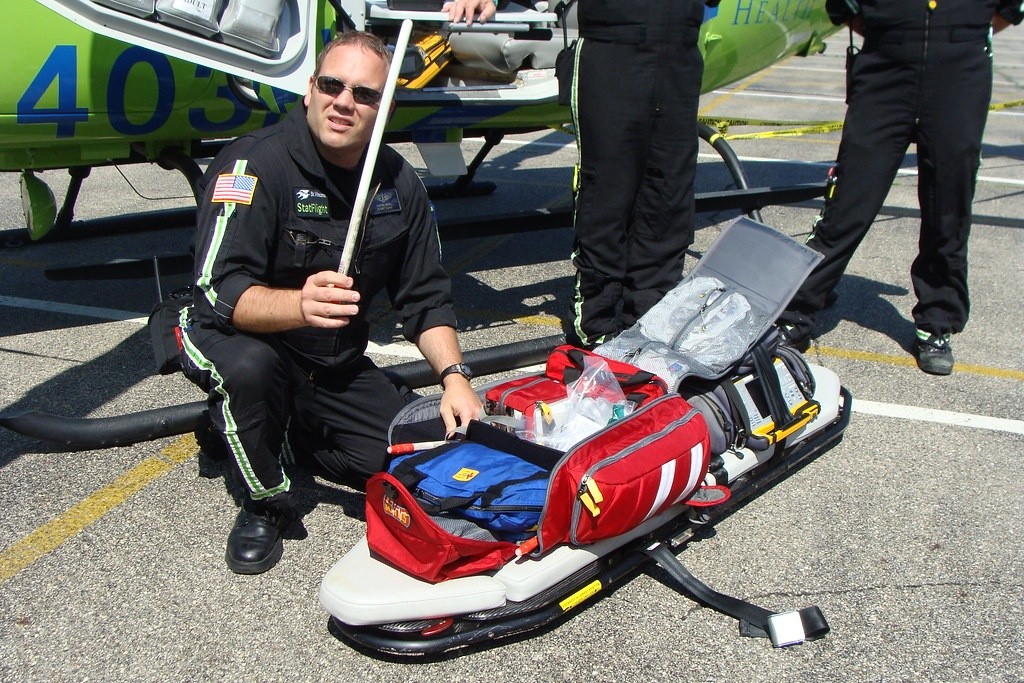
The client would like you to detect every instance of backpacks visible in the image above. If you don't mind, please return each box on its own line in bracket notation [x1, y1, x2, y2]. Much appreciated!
[364, 216, 825, 584]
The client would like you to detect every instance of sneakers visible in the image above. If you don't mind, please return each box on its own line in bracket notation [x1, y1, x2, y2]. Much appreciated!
[915, 334, 954, 375]
[771, 321, 812, 354]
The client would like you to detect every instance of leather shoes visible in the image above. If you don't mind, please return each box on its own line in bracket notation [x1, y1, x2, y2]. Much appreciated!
[225, 494, 298, 575]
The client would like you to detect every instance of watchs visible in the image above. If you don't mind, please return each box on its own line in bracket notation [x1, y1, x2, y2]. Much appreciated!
[441, 361, 474, 390]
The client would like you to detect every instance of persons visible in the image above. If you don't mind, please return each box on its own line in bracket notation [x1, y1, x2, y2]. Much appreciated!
[443, 0, 719, 355]
[775, 0, 1024, 374]
[179, 30, 486, 574]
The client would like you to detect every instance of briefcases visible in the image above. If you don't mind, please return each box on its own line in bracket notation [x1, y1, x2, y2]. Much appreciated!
[375, 29, 454, 89]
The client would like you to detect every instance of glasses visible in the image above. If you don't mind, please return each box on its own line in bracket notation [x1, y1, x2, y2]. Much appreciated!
[312, 77, 382, 105]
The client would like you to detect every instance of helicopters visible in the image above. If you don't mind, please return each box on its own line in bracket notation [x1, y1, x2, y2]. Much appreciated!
[0, 0, 847, 450]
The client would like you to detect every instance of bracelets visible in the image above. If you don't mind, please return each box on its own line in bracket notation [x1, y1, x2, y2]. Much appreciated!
[494, 0, 498, 8]
